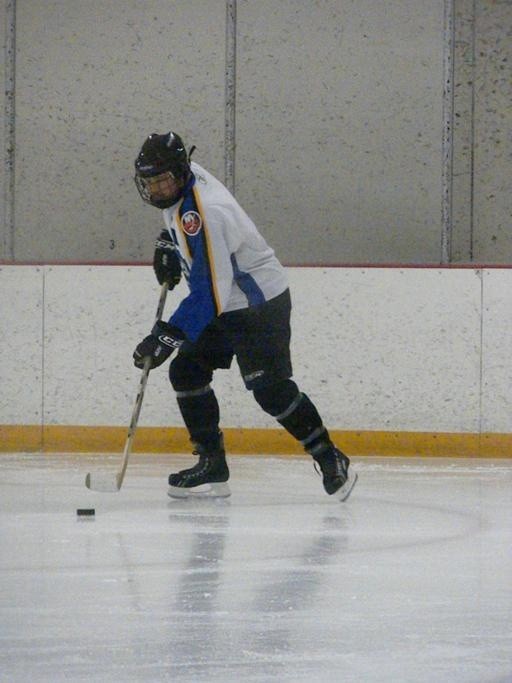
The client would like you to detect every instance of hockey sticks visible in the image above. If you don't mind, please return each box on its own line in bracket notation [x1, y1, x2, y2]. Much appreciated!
[85, 281, 168, 493]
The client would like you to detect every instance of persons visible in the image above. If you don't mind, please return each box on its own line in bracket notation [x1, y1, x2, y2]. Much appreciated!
[132, 131, 350, 495]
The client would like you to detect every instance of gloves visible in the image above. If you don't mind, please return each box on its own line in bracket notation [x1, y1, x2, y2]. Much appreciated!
[154, 229, 182, 290]
[133, 319, 183, 370]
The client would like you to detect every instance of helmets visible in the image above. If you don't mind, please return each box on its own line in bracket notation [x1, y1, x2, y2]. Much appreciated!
[133, 131, 188, 209]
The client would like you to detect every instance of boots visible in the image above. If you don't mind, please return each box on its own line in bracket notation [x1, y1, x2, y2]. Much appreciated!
[169, 432, 229, 487]
[305, 435, 349, 495]
